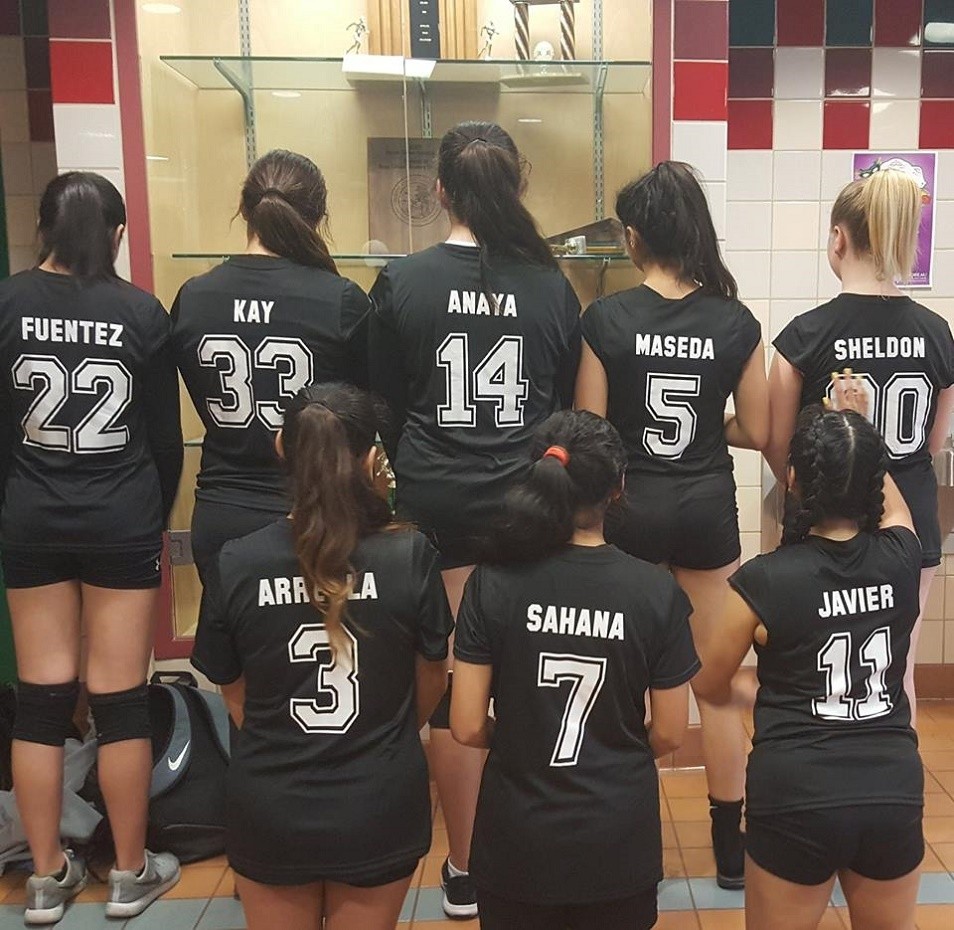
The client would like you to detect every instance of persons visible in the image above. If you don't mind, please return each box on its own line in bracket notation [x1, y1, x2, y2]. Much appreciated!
[345, 18, 370, 54]
[167, 150, 392, 903]
[573, 160, 771, 892]
[475, 20, 500, 59]
[0, 170, 184, 928]
[190, 381, 456, 930]
[697, 367, 927, 930]
[447, 408, 704, 930]
[366, 121, 583, 923]
[761, 158, 954, 730]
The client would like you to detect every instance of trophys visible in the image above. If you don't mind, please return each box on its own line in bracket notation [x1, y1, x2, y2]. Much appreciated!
[500, 0, 590, 88]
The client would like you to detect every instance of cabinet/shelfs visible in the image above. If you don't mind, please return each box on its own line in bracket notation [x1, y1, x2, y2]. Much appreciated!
[161, 54, 654, 449]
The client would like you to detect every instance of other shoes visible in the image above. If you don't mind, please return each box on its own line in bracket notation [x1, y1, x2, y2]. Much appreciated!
[716, 832, 747, 890]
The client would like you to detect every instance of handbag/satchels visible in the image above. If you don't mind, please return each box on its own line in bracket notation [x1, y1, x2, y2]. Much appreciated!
[72, 671, 240, 883]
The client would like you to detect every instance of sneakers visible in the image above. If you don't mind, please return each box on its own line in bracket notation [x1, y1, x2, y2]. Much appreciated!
[439, 854, 478, 916]
[105, 848, 181, 917]
[24, 848, 89, 924]
